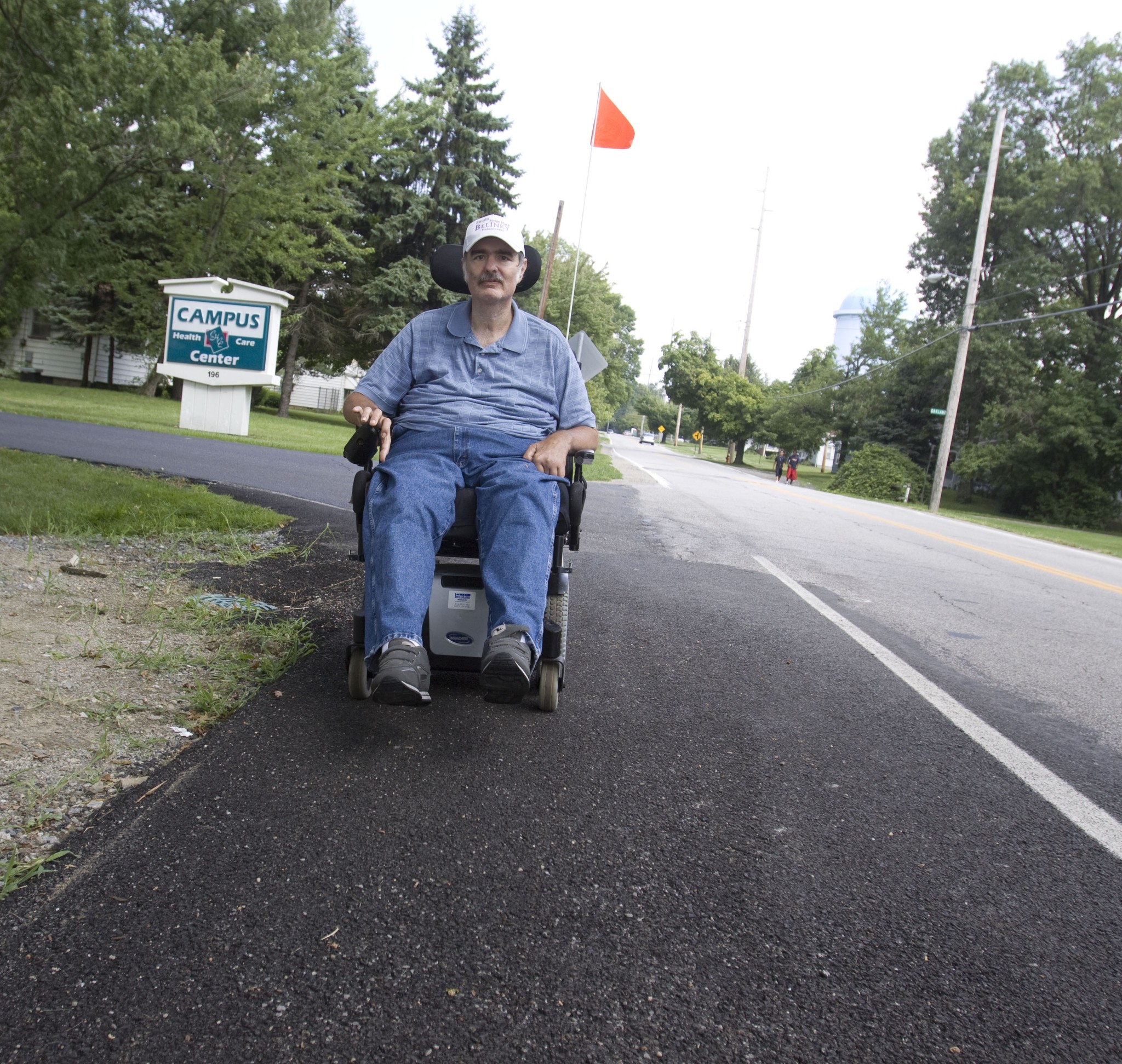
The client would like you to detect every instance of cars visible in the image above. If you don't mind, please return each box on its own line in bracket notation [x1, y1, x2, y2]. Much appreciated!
[607, 429, 613, 434]
[639, 433, 656, 446]
[623, 430, 632, 436]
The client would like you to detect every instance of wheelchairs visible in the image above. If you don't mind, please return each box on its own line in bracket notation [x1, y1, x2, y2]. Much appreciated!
[338, 417, 597, 714]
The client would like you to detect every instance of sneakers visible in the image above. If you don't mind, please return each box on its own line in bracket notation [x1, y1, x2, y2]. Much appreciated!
[371, 639, 432, 707]
[478, 623, 531, 704]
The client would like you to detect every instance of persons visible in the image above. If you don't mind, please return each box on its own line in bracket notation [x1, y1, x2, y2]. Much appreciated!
[342, 214, 600, 703]
[772, 449, 799, 485]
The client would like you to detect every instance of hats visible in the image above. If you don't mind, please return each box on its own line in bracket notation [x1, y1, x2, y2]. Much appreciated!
[463, 214, 525, 257]
